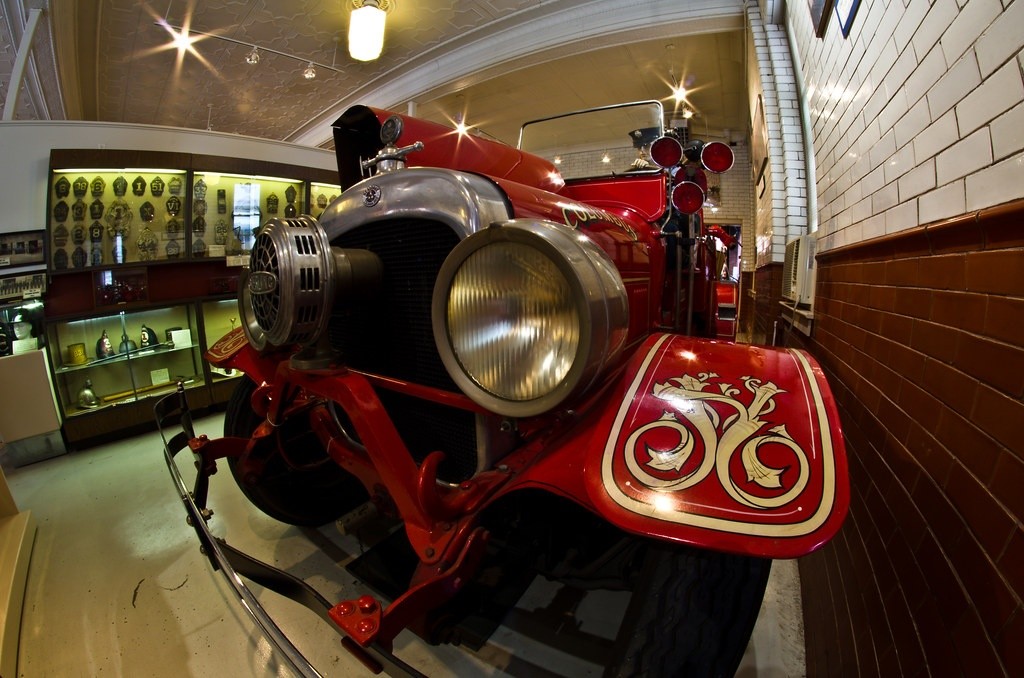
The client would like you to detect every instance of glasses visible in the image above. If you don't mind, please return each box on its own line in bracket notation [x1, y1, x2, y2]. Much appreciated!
[638, 141, 653, 150]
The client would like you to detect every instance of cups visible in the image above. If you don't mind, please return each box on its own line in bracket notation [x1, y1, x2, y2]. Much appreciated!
[66, 344, 87, 364]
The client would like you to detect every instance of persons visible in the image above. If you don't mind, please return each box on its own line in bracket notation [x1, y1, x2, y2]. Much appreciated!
[8, 309, 33, 339]
[628, 126, 685, 272]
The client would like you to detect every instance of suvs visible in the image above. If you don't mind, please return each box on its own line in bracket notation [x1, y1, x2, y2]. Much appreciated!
[150, 100, 851, 678]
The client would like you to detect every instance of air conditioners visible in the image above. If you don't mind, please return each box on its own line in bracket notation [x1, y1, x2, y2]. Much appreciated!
[781, 236, 816, 303]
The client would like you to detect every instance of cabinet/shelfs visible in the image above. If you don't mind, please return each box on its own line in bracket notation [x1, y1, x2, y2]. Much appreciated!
[42, 148, 342, 452]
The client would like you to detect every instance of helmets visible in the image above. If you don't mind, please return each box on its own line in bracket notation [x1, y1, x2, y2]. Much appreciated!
[75, 379, 101, 408]
[7, 307, 34, 324]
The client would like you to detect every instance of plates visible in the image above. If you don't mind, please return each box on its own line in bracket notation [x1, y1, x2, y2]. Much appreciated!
[64, 359, 95, 366]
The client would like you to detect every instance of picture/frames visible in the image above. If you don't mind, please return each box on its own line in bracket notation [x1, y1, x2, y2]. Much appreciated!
[750, 94, 769, 186]
[807, 0, 833, 38]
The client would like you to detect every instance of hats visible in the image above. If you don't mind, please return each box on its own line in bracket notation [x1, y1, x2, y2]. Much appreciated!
[64, 343, 93, 366]
[628, 126, 661, 148]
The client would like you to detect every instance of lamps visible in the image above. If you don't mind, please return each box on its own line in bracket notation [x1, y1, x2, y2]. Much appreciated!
[348, 0, 390, 61]
[153, 0, 345, 79]
